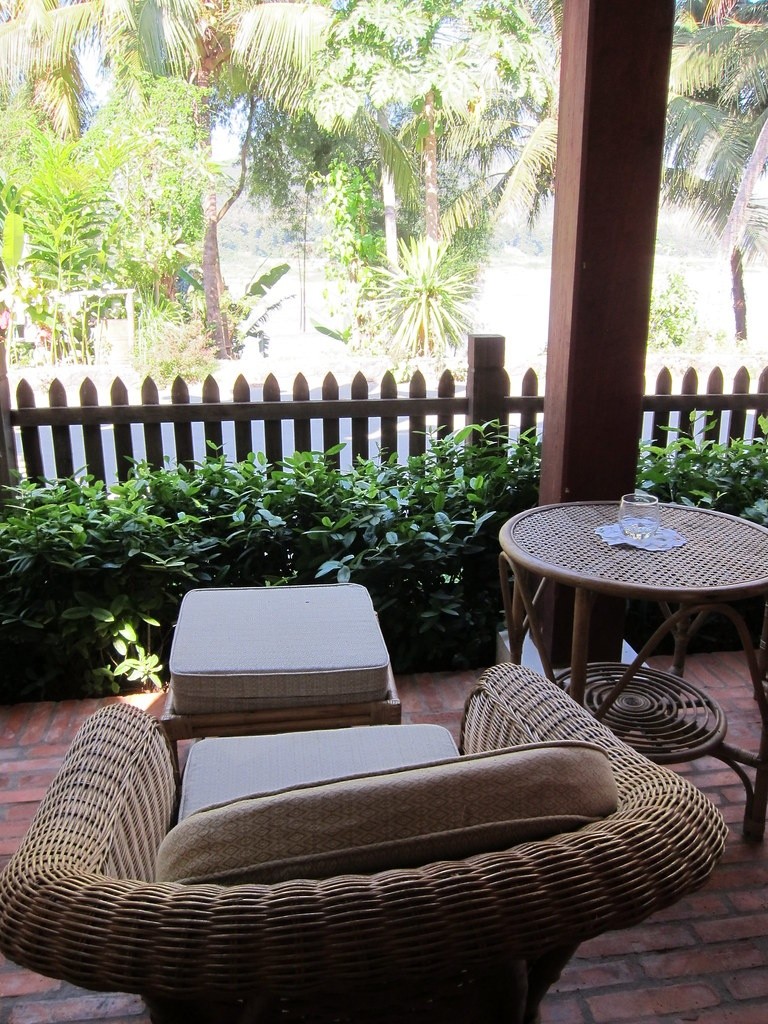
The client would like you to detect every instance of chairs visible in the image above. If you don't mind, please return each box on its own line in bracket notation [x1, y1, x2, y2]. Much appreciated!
[1, 661, 731, 1022]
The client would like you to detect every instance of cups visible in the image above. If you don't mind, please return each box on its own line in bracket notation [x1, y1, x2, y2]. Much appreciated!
[619, 493, 660, 548]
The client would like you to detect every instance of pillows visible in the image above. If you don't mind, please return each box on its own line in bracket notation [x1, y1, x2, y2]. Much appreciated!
[154, 739, 620, 888]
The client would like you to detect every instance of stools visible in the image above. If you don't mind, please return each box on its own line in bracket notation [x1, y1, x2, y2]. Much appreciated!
[158, 581, 401, 774]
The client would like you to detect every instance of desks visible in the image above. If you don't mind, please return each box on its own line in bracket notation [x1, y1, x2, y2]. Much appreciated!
[499, 500, 768, 842]
